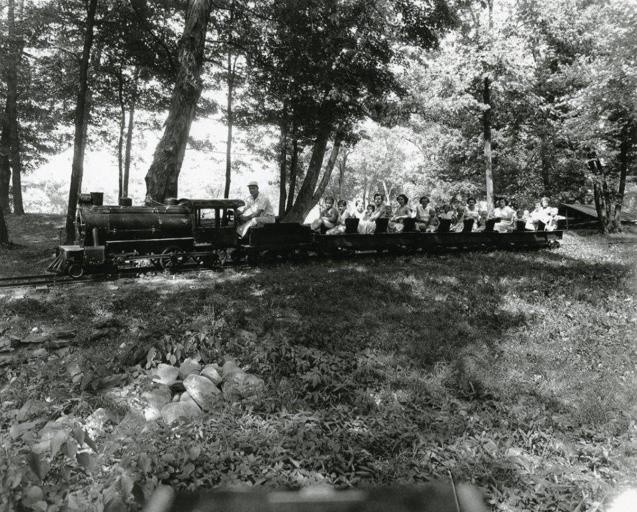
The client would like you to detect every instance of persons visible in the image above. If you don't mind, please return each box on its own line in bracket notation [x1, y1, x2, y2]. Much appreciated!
[235, 181, 275, 241]
[310, 193, 560, 235]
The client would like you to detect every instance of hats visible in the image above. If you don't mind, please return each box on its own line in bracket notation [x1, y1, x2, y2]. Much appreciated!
[247, 182, 257, 186]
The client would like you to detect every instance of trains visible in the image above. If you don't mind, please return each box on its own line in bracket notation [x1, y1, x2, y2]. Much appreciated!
[47, 192, 563, 280]
